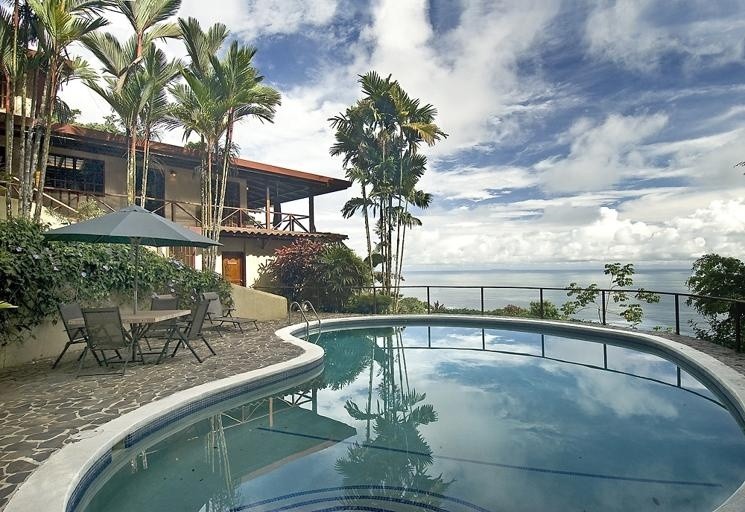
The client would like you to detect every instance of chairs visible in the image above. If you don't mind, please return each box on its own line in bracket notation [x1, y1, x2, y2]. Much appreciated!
[51, 295, 217, 377]
[201, 291, 259, 337]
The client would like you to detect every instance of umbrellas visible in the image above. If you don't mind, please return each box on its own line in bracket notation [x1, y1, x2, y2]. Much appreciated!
[41, 201, 223, 309]
